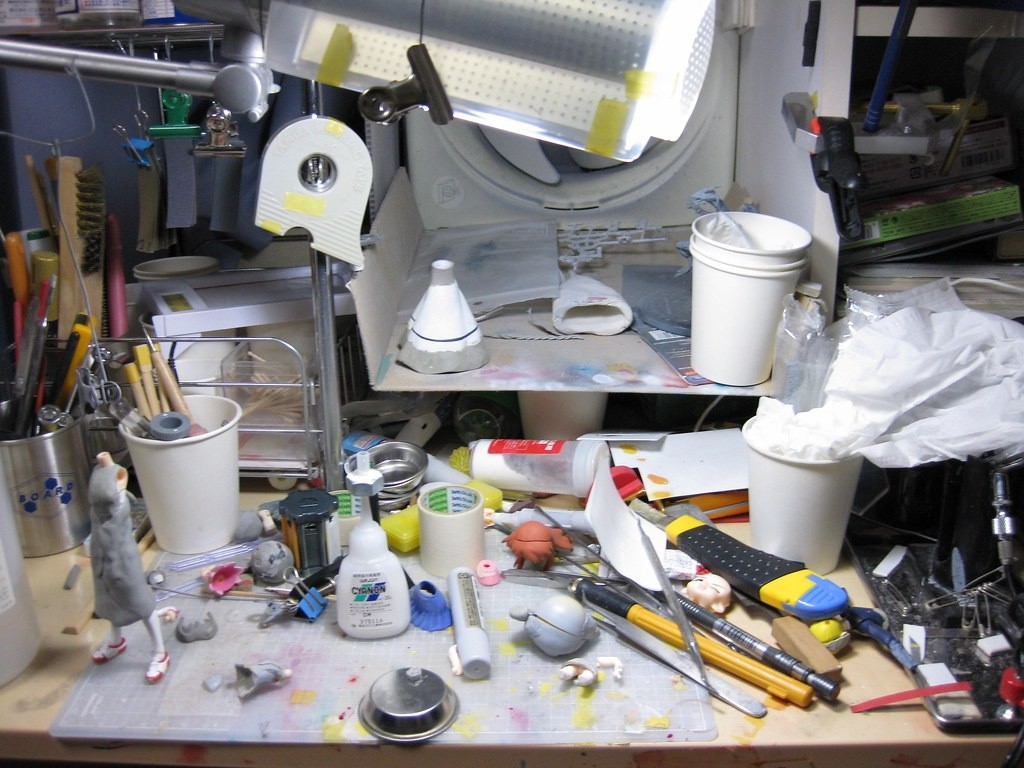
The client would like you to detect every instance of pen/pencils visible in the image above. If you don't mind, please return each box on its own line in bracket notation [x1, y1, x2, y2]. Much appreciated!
[2, 274, 58, 439]
[646, 587, 841, 702]
[568, 577, 814, 707]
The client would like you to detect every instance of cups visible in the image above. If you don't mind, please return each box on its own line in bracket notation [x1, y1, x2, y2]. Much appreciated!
[139, 311, 237, 402]
[690, 211, 813, 388]
[0, 396, 90, 558]
[0, 465, 42, 687]
[133, 256, 216, 283]
[119, 395, 243, 554]
[742, 415, 865, 576]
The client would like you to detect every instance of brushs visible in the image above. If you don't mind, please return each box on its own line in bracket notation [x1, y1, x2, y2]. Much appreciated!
[57, 155, 104, 349]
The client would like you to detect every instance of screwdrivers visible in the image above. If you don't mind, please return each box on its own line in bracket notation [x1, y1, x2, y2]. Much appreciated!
[109, 398, 156, 441]
[141, 325, 208, 439]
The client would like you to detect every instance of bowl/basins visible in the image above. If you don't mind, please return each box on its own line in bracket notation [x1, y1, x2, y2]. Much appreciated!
[344, 441, 428, 510]
[358, 669, 460, 742]
